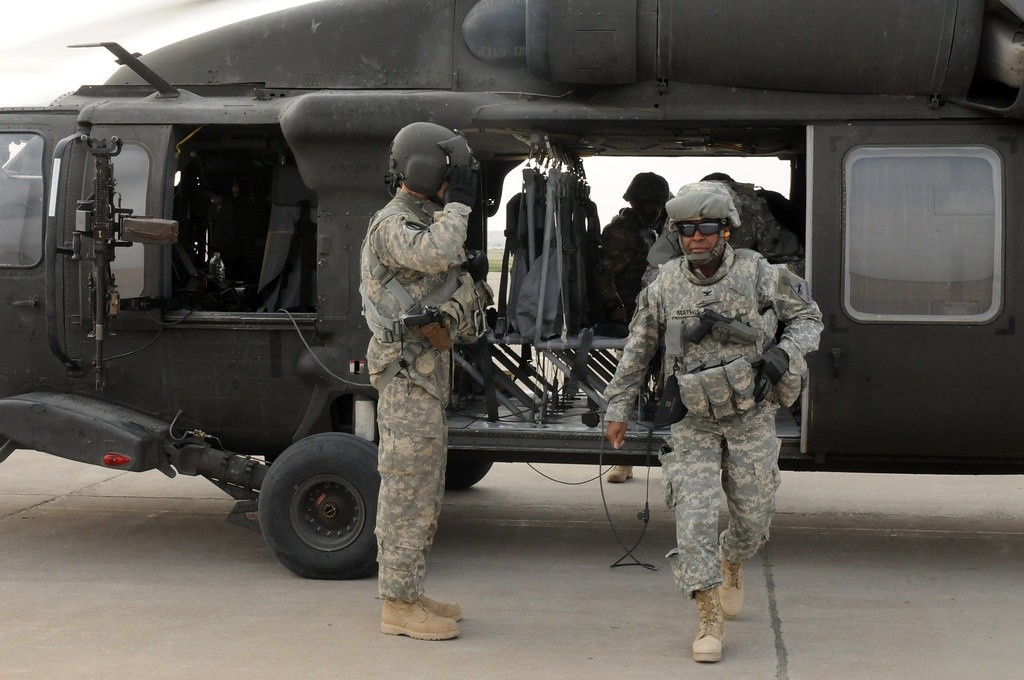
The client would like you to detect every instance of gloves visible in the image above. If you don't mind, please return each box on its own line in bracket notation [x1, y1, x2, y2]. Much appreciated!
[444, 166, 478, 206]
[751, 347, 789, 403]
[466, 250, 488, 278]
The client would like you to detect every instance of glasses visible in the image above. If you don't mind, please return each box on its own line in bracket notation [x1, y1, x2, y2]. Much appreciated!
[677, 223, 729, 237]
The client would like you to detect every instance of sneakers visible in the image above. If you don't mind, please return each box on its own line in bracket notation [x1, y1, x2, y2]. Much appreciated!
[607, 464, 633, 482]
[381, 595, 463, 640]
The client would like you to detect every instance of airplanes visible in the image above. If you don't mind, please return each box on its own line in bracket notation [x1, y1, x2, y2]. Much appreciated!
[1, 1, 1023, 579]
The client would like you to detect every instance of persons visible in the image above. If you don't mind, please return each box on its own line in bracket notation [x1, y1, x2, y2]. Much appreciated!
[602, 181, 826, 662]
[598, 170, 805, 483]
[359, 121, 482, 641]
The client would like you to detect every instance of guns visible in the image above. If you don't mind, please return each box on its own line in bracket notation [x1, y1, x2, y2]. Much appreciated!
[686, 308, 732, 346]
[402, 305, 444, 329]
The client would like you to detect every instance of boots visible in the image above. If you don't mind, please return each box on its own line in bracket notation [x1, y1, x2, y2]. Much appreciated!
[693, 586, 726, 663]
[718, 547, 744, 620]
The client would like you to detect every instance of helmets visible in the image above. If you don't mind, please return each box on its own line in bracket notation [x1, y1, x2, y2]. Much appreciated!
[665, 182, 741, 228]
[389, 121, 470, 196]
[622, 172, 671, 204]
